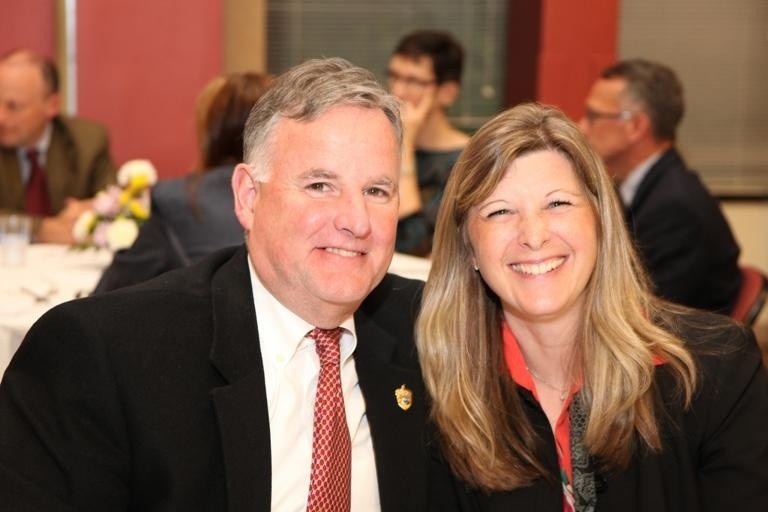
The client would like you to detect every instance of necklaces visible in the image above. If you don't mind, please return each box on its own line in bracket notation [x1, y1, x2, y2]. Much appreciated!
[527, 368, 568, 401]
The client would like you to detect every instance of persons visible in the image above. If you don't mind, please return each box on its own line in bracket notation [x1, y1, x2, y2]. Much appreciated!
[364, 26, 489, 262]
[1, 55, 463, 511]
[380, 99, 766, 511]
[567, 55, 743, 313]
[91, 65, 286, 298]
[0, 44, 120, 252]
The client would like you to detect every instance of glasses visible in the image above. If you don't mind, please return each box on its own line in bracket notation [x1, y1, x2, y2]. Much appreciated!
[582, 106, 633, 126]
[380, 65, 439, 97]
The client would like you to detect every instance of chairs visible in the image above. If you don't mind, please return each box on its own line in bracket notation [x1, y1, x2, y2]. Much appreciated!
[729, 262, 768, 326]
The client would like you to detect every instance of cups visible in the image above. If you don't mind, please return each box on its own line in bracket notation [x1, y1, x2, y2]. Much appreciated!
[0, 215, 30, 263]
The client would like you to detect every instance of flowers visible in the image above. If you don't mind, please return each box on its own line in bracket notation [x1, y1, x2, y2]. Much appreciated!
[72, 160, 158, 271]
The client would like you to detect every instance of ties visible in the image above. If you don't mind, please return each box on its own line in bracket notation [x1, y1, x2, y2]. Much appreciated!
[306, 325, 353, 512]
[23, 147, 54, 216]
